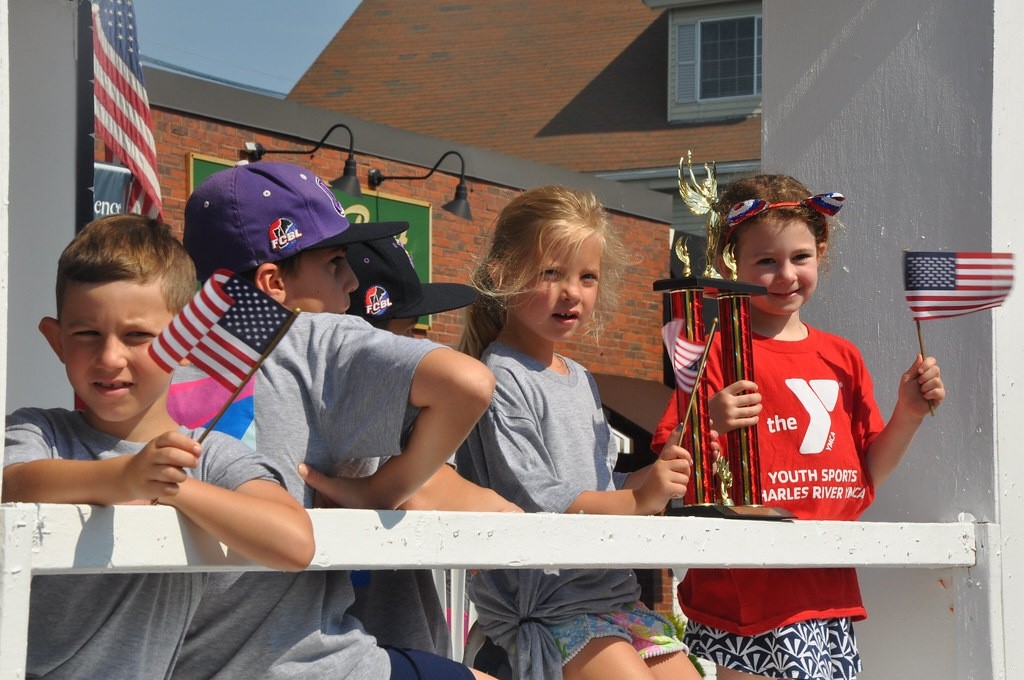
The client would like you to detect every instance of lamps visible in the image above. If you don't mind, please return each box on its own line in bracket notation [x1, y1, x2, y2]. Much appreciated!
[241, 121, 363, 201]
[367, 150, 475, 226]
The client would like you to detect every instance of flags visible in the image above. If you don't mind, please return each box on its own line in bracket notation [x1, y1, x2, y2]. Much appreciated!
[904, 251, 1016, 322]
[92, 0, 162, 226]
[660, 320, 705, 393]
[149, 269, 296, 396]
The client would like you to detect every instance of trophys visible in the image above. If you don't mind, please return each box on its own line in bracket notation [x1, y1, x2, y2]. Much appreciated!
[653, 150, 799, 519]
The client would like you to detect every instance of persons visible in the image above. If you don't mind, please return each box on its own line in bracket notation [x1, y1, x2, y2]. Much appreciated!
[457, 184, 703, 680]
[3, 213, 316, 680]
[653, 176, 945, 680]
[184, 160, 496, 680]
[347, 238, 527, 657]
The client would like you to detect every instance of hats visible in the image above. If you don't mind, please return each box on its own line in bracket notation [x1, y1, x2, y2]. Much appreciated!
[183, 160, 410, 281]
[343, 236, 480, 322]
